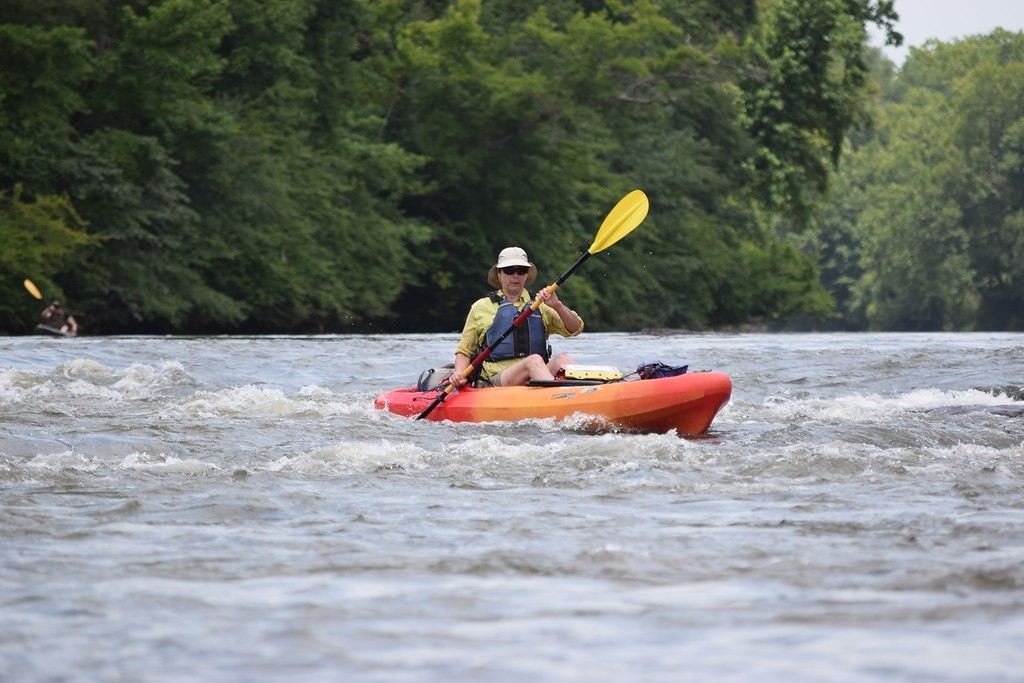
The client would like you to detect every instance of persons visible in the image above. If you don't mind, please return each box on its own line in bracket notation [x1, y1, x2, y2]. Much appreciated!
[448, 247, 584, 387]
[41, 300, 78, 333]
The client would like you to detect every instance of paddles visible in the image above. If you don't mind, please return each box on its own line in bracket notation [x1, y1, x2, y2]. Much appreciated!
[420, 188, 650, 422]
[23, 278, 68, 325]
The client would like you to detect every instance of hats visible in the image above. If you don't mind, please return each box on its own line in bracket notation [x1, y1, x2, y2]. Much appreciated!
[488, 247, 537, 289]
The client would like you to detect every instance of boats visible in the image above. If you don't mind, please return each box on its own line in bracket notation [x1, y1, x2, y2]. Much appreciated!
[373, 373, 732, 437]
[34, 323, 77, 337]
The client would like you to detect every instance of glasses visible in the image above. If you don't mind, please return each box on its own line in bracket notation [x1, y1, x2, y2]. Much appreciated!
[501, 266, 529, 275]
